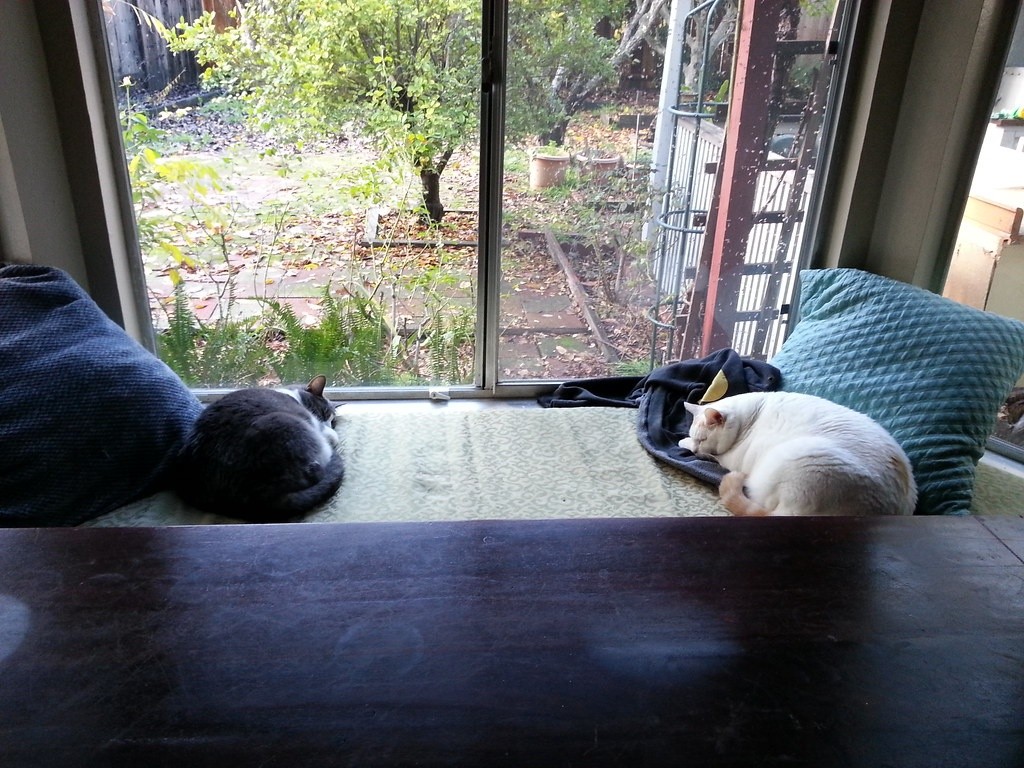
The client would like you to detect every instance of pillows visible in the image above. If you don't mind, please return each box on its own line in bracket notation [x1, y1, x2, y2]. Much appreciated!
[768, 267, 1024, 515]
[0, 263, 205, 528]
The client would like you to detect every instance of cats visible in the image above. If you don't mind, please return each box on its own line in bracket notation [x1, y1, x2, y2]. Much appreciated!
[175, 374, 346, 524]
[678, 391, 918, 517]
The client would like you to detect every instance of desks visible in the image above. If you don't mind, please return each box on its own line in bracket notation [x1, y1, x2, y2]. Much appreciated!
[0, 514, 1024, 768]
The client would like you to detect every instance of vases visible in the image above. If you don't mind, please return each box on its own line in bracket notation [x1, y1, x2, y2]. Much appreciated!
[528, 145, 569, 189]
[578, 149, 618, 184]
